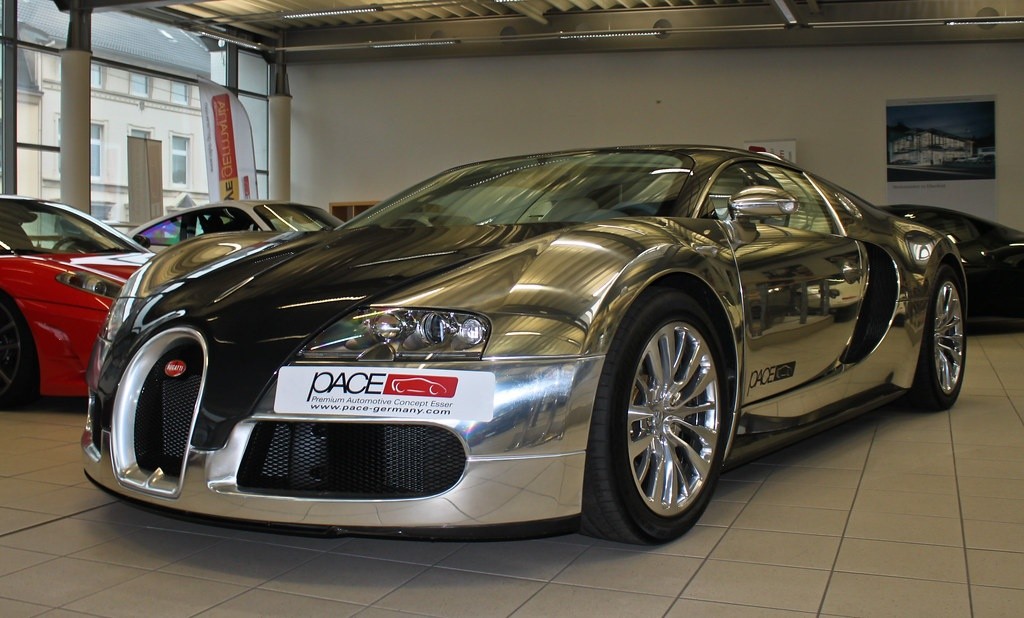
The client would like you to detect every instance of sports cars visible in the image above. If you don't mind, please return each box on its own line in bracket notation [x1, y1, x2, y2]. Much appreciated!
[0, 195, 156, 410]
[81, 144, 970, 545]
[126, 200, 346, 254]
[878, 204, 1024, 330]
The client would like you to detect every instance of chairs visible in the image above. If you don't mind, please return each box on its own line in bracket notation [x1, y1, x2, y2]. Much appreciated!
[536, 194, 600, 225]
[204, 214, 227, 233]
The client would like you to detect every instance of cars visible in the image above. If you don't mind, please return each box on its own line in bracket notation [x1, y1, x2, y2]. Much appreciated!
[895, 291, 912, 328]
[716, 290, 746, 343]
[795, 270, 925, 310]
[737, 277, 806, 319]
